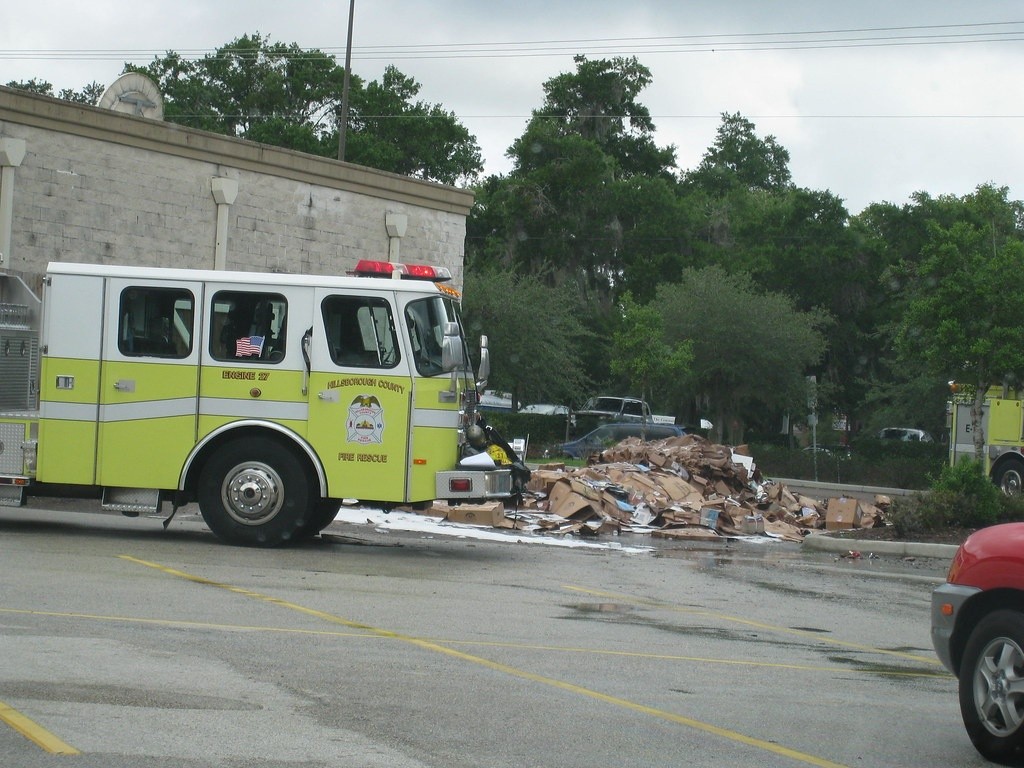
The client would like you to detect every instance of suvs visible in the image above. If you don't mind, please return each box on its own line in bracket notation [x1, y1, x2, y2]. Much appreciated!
[569, 397, 653, 436]
[541, 424, 686, 461]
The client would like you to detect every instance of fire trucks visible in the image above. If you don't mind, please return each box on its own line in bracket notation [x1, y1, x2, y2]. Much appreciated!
[946, 382, 1023, 500]
[1, 260, 532, 547]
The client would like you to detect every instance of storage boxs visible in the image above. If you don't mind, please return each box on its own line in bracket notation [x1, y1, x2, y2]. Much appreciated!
[826, 496, 863, 531]
[447, 501, 505, 526]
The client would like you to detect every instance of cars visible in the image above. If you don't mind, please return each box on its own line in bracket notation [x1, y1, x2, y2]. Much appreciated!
[932, 520, 1024, 767]
